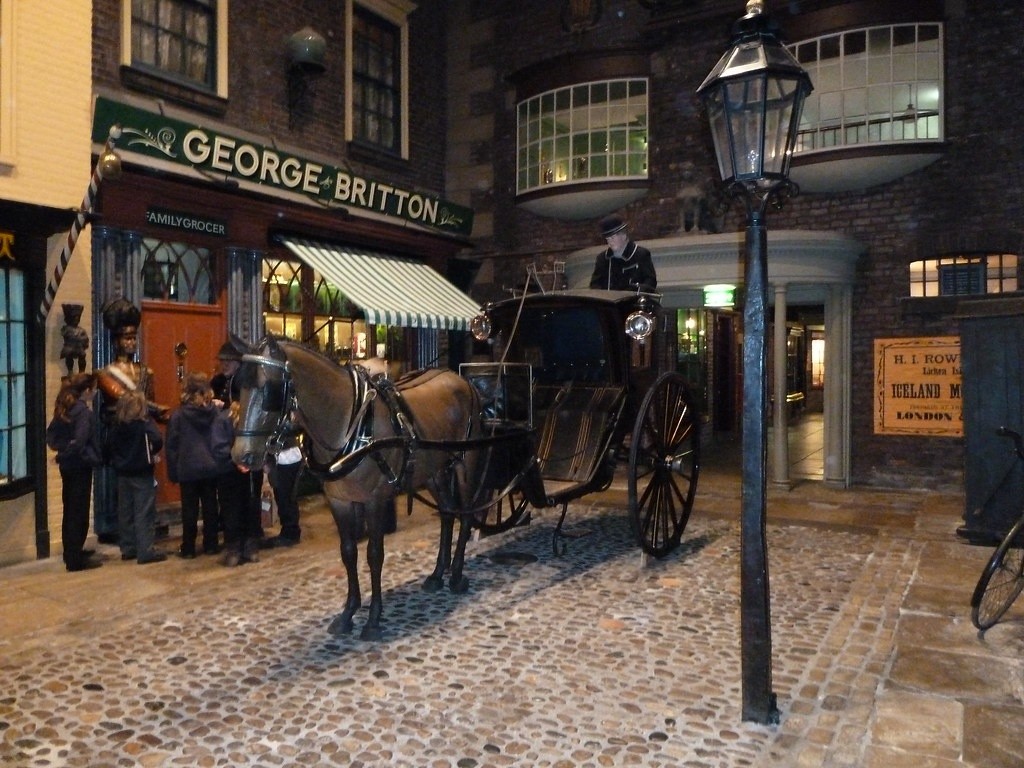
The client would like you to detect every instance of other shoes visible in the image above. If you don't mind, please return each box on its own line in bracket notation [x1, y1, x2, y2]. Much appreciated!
[225, 551, 241, 566]
[98, 534, 120, 544]
[204, 546, 217, 554]
[242, 547, 259, 562]
[268, 536, 299, 546]
[80, 550, 96, 558]
[175, 550, 195, 559]
[121, 550, 137, 560]
[137, 553, 167, 564]
[68, 558, 103, 571]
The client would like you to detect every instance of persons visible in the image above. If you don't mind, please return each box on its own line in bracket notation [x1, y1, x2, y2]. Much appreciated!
[94, 299, 172, 545]
[45, 372, 106, 572]
[58, 302, 89, 374]
[206, 344, 306, 568]
[106, 388, 166, 564]
[589, 212, 659, 294]
[164, 367, 224, 558]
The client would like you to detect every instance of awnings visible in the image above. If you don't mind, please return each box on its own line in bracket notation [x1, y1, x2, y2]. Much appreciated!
[278, 229, 489, 334]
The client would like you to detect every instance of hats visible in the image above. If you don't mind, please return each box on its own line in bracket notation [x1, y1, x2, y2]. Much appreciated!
[596, 213, 628, 239]
[215, 342, 242, 362]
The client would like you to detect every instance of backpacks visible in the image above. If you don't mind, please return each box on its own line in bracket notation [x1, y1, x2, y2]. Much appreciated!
[45, 406, 89, 451]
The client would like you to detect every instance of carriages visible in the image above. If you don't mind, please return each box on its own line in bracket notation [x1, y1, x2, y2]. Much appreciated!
[227, 255, 702, 636]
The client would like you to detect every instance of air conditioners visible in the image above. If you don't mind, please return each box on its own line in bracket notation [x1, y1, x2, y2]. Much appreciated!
[938, 262, 985, 296]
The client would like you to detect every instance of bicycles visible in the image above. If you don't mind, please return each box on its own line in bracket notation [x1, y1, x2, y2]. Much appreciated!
[970, 426, 1024, 631]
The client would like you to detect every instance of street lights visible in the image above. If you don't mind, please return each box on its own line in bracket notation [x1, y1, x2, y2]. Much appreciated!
[690, 1, 816, 727]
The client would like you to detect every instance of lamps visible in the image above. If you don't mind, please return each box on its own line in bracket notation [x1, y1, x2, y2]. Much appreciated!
[624, 311, 657, 341]
[472, 315, 500, 341]
[903, 84, 919, 123]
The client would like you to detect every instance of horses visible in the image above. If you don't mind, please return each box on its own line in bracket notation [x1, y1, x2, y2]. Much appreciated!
[223, 326, 487, 642]
